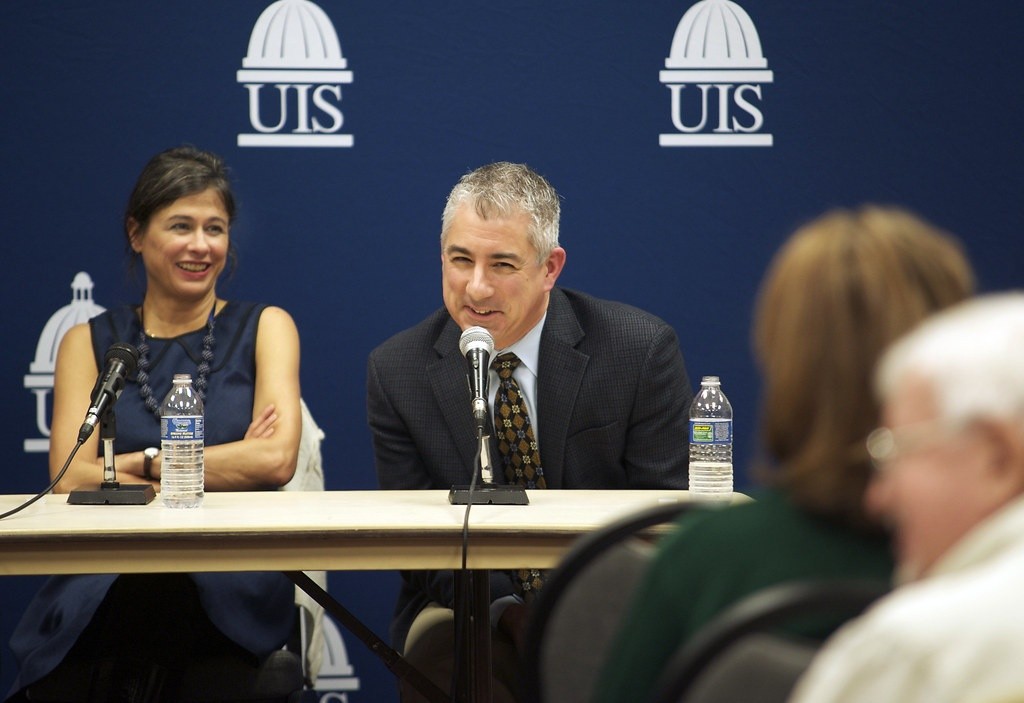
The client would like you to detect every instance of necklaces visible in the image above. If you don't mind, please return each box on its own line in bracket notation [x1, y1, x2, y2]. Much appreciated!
[137, 297, 217, 420]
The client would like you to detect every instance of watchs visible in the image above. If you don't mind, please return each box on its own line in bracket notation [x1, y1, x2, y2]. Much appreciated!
[143, 447, 159, 480]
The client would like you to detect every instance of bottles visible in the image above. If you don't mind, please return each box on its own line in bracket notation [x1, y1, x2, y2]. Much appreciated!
[689, 376, 733, 505]
[160, 374, 205, 510]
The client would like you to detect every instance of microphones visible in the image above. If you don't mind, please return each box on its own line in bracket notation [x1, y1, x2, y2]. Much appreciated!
[459, 326, 495, 429]
[76, 342, 140, 445]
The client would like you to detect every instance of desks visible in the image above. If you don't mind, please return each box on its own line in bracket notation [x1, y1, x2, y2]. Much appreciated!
[0, 486, 755, 575]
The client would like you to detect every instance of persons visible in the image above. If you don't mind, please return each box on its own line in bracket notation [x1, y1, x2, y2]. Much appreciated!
[788, 292, 1024, 703]
[593, 207, 979, 703]
[368, 162, 694, 703]
[5, 151, 302, 703]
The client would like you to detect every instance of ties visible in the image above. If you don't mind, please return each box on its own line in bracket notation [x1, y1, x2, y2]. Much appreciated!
[490, 352, 555, 606]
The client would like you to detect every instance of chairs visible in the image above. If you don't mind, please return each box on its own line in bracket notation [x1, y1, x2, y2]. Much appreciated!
[283, 397, 361, 703]
